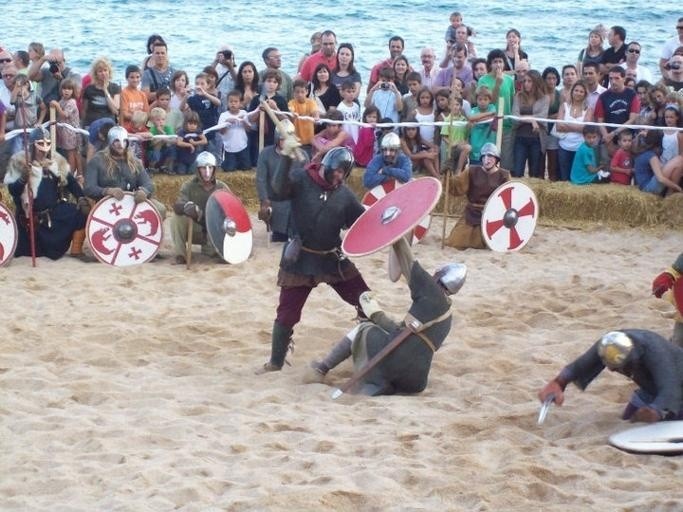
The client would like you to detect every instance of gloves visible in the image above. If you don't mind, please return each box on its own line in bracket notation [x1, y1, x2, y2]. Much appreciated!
[102, 187, 124, 201]
[538, 382, 565, 407]
[184, 201, 203, 223]
[280, 135, 303, 155]
[77, 196, 91, 215]
[258, 199, 272, 224]
[133, 189, 147, 204]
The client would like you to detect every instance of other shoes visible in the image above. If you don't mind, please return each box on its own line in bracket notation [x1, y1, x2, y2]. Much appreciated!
[170, 255, 186, 265]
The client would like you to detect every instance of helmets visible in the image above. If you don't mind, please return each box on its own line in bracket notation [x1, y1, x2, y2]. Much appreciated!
[274, 119, 296, 145]
[195, 151, 217, 167]
[107, 126, 130, 149]
[321, 146, 355, 184]
[380, 131, 401, 150]
[435, 263, 469, 294]
[29, 126, 51, 153]
[480, 143, 500, 162]
[598, 331, 634, 367]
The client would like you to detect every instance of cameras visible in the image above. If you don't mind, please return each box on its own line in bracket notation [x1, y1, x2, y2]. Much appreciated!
[48, 60, 60, 74]
[663, 63, 680, 70]
[223, 50, 232, 60]
[379, 82, 392, 90]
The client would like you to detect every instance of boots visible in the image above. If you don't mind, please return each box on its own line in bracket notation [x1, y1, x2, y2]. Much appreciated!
[312, 336, 351, 375]
[259, 322, 293, 374]
[71, 230, 86, 259]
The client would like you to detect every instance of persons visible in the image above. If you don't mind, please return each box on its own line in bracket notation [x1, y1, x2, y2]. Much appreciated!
[256, 118, 310, 241]
[447, 142, 511, 251]
[0, 11, 682, 198]
[308, 237, 467, 395]
[252, 136, 373, 376]
[537, 328, 683, 423]
[5, 126, 90, 261]
[363, 132, 413, 188]
[170, 150, 233, 265]
[83, 126, 166, 261]
[651, 253, 683, 350]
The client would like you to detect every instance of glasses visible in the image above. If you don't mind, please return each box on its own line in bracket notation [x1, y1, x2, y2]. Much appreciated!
[627, 49, 640, 53]
[0, 58, 11, 63]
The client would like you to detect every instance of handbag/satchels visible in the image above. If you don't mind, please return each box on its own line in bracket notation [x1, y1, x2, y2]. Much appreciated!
[550, 103, 568, 139]
